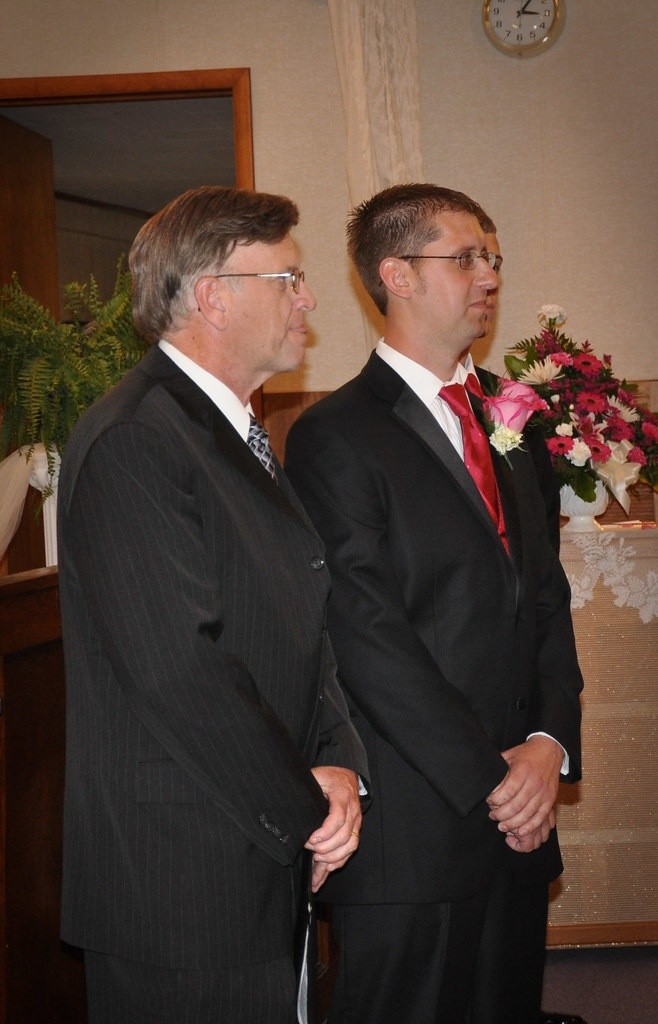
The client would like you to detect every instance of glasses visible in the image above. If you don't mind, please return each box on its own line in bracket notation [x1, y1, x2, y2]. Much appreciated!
[376, 251, 496, 288]
[198, 267, 304, 313]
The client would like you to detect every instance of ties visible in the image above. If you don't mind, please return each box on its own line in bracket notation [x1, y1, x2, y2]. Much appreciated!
[464, 373, 489, 402]
[247, 413, 280, 489]
[438, 384, 510, 559]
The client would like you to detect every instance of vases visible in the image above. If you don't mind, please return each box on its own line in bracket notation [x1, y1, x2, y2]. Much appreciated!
[559, 479, 610, 532]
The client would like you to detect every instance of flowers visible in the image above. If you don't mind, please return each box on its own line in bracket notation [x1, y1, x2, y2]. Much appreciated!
[499, 302, 658, 514]
[485, 376, 551, 470]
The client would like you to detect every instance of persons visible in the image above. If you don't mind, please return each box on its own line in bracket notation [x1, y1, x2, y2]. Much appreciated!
[284, 182, 581, 1024]
[55, 185, 372, 1024]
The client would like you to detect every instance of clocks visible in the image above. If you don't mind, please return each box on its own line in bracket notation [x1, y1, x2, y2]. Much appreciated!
[482, 0, 567, 59]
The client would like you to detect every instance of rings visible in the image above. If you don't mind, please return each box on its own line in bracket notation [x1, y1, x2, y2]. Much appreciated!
[350, 832, 360, 837]
